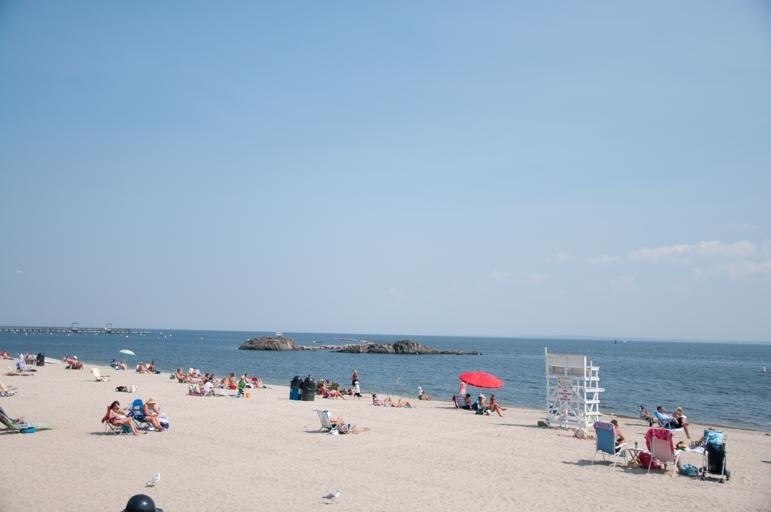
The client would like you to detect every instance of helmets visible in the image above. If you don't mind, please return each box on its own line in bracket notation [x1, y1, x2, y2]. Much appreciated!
[122, 494, 162, 511]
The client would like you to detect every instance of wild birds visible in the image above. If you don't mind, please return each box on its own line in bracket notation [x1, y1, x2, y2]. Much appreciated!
[321, 489, 340, 504]
[144, 472, 160, 488]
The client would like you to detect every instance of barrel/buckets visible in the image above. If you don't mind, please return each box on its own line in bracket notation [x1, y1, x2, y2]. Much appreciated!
[290, 380, 302, 400]
[302, 382, 315, 401]
[246, 391, 252, 398]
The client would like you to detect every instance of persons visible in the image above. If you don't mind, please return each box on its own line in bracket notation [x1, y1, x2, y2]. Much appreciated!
[320, 369, 360, 400]
[323, 411, 343, 429]
[605, 403, 730, 457]
[65, 356, 86, 370]
[0, 406, 21, 430]
[0, 353, 37, 397]
[417, 385, 432, 400]
[459, 381, 507, 417]
[110, 358, 156, 374]
[142, 397, 164, 432]
[175, 367, 267, 398]
[373, 394, 417, 408]
[108, 400, 140, 435]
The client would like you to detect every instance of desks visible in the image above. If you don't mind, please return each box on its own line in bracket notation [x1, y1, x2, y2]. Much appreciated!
[627, 448, 644, 468]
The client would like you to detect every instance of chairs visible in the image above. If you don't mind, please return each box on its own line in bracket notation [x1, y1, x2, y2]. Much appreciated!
[646, 427, 681, 478]
[457, 395, 467, 408]
[314, 407, 345, 433]
[102, 398, 170, 436]
[92, 367, 110, 382]
[590, 420, 628, 469]
[654, 416, 687, 439]
[699, 432, 731, 483]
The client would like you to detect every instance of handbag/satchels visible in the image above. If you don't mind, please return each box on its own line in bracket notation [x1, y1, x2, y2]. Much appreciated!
[639, 451, 657, 468]
[681, 464, 698, 476]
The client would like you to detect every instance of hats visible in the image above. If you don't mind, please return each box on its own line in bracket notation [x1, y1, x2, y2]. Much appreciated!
[146, 398, 157, 404]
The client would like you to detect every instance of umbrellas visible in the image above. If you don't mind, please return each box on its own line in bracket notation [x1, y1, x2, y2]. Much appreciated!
[119, 349, 136, 364]
